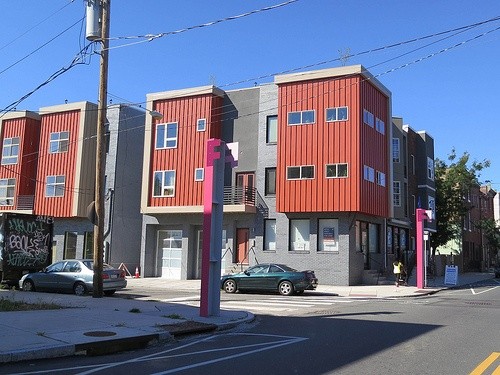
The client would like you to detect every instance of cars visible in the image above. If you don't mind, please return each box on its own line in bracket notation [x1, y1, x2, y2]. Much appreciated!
[221, 263, 318, 296]
[19, 259, 127, 296]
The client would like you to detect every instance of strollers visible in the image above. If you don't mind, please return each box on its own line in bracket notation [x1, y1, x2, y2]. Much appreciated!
[398, 266, 408, 286]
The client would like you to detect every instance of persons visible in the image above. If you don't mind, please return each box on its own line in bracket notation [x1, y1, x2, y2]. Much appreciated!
[392, 257, 407, 287]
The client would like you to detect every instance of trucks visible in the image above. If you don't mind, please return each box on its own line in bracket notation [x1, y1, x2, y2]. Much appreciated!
[0, 212, 54, 289]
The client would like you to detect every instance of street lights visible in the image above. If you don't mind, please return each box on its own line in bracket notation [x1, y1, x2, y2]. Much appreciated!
[93, 91, 164, 303]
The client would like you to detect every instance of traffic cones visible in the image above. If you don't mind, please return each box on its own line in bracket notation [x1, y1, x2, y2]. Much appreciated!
[132, 267, 141, 278]
